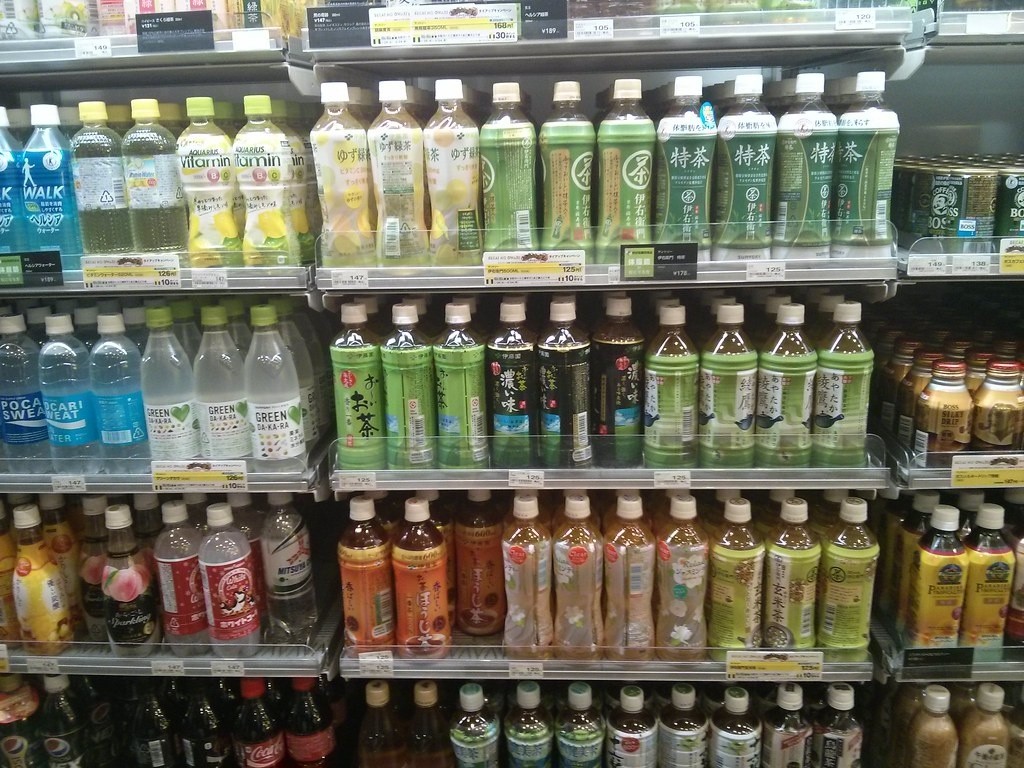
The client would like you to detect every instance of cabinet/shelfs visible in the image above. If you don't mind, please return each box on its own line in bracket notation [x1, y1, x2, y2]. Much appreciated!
[0, 0, 1023, 684]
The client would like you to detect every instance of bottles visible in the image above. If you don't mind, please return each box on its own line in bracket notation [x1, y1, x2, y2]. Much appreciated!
[551, 489, 604, 660]
[537, 292, 593, 469]
[311, 80, 483, 266]
[329, 294, 537, 469]
[480, 82, 539, 251]
[871, 304, 1024, 469]
[338, 490, 551, 661]
[592, 291, 642, 467]
[881, 487, 1024, 660]
[604, 490, 880, 662]
[540, 70, 900, 265]
[0, 492, 318, 656]
[358, 679, 655, 768]
[874, 682, 1024, 768]
[0, 294, 329, 473]
[0, 672, 348, 768]
[0, 95, 314, 268]
[0, 0, 820, 43]
[644, 286, 875, 468]
[656, 682, 862, 768]
